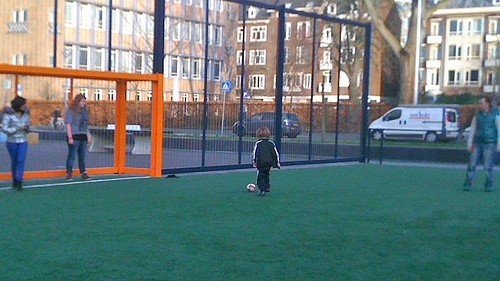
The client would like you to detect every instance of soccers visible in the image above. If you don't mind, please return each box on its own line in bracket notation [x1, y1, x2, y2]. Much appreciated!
[247, 183, 256, 192]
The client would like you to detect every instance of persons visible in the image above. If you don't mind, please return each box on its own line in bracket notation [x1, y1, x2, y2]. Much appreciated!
[65, 93, 92, 182]
[251, 126, 281, 196]
[2, 96, 31, 192]
[462, 95, 500, 195]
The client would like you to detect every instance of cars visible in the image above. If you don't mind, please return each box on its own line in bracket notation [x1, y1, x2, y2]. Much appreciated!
[461, 128, 472, 144]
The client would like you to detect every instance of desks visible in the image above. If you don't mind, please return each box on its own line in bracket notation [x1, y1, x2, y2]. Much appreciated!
[88, 128, 173, 154]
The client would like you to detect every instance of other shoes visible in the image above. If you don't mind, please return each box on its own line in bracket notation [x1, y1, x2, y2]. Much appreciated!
[12, 181, 24, 191]
[485, 186, 493, 191]
[81, 173, 91, 180]
[257, 191, 265, 196]
[464, 185, 472, 191]
[265, 188, 270, 193]
[66, 175, 73, 182]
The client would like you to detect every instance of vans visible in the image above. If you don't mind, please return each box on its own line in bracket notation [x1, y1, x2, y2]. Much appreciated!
[368, 107, 460, 142]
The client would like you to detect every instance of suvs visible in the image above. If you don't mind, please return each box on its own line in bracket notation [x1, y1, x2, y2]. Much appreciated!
[232, 112, 303, 138]
